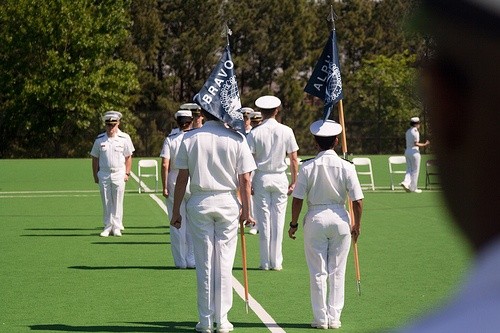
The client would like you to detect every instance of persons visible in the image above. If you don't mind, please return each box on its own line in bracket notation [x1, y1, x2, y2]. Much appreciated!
[90, 110, 136, 237]
[390, 0, 500, 333]
[401, 117, 430, 194]
[245, 95, 300, 271]
[169, 93, 258, 333]
[160, 110, 196, 270]
[237, 107, 265, 236]
[169, 102, 205, 136]
[287, 119, 364, 328]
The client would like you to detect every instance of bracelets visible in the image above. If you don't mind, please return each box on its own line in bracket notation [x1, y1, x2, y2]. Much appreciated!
[290, 221, 299, 228]
[126, 173, 130, 176]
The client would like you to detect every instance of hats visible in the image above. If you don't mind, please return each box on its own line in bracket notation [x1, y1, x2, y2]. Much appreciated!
[409, 116, 420, 123]
[310, 120, 342, 138]
[104, 115, 118, 124]
[251, 112, 263, 121]
[241, 108, 254, 118]
[256, 96, 281, 112]
[180, 103, 202, 116]
[176, 111, 192, 122]
[105, 111, 122, 118]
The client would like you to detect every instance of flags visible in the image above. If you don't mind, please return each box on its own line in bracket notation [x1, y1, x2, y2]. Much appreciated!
[303, 28, 346, 125]
[192, 44, 245, 136]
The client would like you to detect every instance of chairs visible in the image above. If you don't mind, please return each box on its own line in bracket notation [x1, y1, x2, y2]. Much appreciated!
[138, 159, 159, 193]
[351, 158, 375, 191]
[425, 159, 441, 190]
[388, 156, 407, 190]
[285, 157, 302, 176]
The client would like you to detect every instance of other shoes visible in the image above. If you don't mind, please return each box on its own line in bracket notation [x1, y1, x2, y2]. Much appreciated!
[112, 229, 122, 237]
[311, 320, 329, 329]
[329, 321, 342, 329]
[250, 229, 258, 235]
[401, 182, 423, 193]
[196, 322, 214, 333]
[216, 323, 233, 333]
[100, 230, 111, 236]
